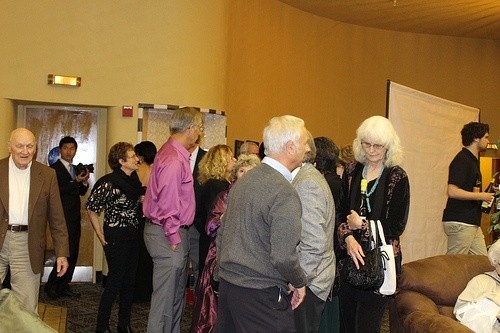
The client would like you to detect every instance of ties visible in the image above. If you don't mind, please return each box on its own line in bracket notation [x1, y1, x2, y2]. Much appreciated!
[69, 163, 77, 180]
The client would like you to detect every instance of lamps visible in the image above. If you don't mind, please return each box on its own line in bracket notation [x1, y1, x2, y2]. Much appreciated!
[48, 74, 81, 86]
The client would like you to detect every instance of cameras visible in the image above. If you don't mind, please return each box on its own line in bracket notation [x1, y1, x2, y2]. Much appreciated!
[74, 162, 94, 175]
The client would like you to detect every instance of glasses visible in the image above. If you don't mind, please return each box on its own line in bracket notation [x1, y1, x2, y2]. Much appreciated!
[363, 142, 386, 150]
[188, 124, 205, 132]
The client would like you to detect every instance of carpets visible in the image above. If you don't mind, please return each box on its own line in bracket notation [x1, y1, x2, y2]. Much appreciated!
[39, 302, 68, 333]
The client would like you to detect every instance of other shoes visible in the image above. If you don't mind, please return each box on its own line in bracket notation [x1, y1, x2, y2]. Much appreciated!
[62, 291, 81, 297]
[45, 284, 62, 302]
[117, 324, 133, 333]
[96, 326, 110, 333]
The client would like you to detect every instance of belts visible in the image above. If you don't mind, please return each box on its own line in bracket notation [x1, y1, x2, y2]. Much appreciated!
[146, 219, 189, 231]
[7, 224, 28, 232]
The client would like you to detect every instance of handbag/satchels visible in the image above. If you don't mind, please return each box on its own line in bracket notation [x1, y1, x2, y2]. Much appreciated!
[343, 220, 397, 295]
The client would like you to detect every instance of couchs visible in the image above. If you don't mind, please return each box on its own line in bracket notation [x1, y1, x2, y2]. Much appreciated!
[388, 254, 494, 333]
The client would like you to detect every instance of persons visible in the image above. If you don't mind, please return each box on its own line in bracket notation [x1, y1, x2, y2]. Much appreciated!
[143, 107, 205, 333]
[439, 122, 495, 256]
[334, 114, 410, 333]
[212, 114, 312, 333]
[133, 141, 157, 306]
[40, 136, 90, 303]
[83, 142, 149, 333]
[184, 129, 359, 332]
[0, 128, 69, 318]
